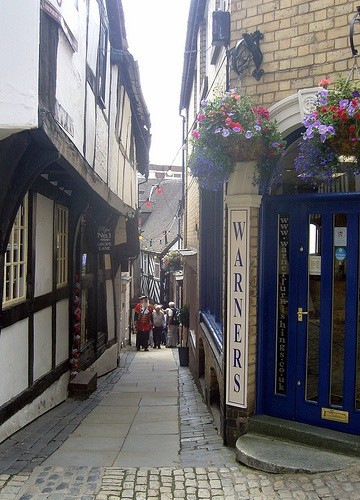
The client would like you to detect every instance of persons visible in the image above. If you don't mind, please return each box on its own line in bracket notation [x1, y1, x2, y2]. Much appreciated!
[148, 301, 179, 349]
[134, 295, 154, 351]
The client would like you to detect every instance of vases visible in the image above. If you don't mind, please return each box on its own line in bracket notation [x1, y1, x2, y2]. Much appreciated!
[220, 136, 264, 162]
[323, 122, 356, 155]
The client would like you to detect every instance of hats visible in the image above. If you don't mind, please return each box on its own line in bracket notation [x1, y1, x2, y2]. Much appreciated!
[169, 301, 175, 307]
[155, 305, 160, 310]
[139, 296, 148, 300]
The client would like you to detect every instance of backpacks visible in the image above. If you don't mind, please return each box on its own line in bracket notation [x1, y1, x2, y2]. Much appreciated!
[173, 312, 180, 325]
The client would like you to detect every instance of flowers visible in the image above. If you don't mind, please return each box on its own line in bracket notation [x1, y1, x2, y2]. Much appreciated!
[185, 74, 288, 197]
[294, 70, 360, 191]
[160, 250, 183, 273]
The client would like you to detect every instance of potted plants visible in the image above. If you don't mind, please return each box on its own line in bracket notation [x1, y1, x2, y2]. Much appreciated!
[172, 304, 191, 366]
